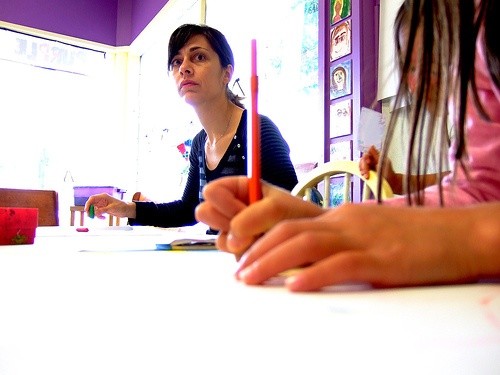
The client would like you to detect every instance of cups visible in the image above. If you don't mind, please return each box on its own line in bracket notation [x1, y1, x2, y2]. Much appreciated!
[1, 207, 38, 245]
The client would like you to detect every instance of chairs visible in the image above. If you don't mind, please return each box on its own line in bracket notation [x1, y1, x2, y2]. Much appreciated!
[290, 161, 395, 208]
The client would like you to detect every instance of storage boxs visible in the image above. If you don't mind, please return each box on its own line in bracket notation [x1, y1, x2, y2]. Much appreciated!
[72, 184, 127, 205]
[0, 185, 60, 244]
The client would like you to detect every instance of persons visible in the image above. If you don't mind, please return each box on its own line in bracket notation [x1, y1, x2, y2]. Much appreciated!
[359, 0, 454, 192]
[193, 0, 500, 291]
[82, 24, 298, 235]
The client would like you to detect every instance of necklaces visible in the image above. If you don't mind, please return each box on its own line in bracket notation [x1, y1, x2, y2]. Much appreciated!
[213, 108, 232, 143]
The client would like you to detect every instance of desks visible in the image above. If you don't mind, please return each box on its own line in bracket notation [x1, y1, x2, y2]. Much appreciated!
[0, 221, 499, 375]
[69, 205, 121, 230]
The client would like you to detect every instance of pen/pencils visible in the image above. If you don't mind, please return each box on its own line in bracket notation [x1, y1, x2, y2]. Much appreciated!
[247, 37, 264, 245]
[157, 241, 216, 251]
